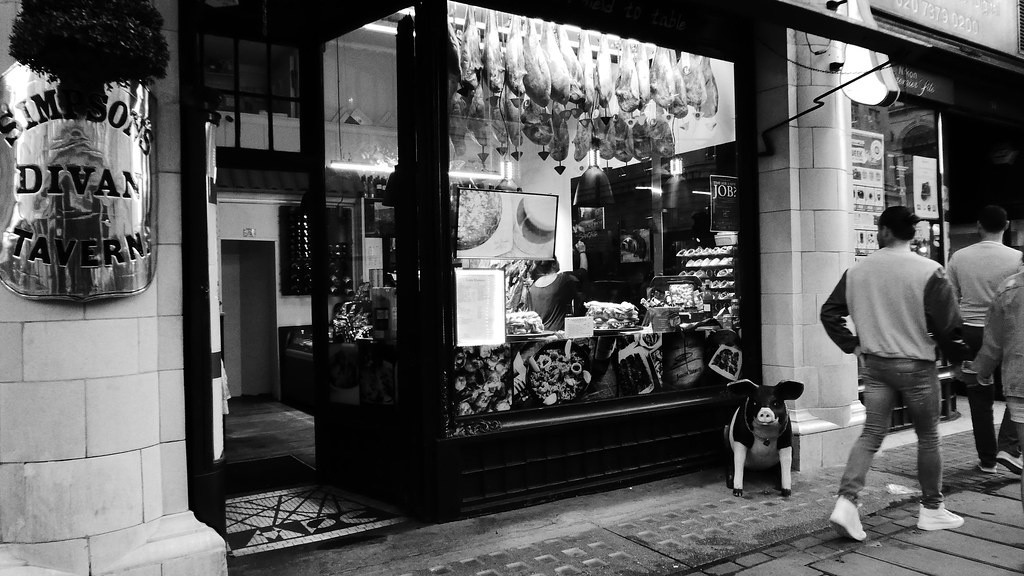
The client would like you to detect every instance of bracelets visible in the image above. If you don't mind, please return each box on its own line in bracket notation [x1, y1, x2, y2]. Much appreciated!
[580, 252, 586, 253]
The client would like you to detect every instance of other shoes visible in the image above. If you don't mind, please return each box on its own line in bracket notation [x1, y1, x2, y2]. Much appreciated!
[995, 450, 1023, 475]
[977, 458, 997, 473]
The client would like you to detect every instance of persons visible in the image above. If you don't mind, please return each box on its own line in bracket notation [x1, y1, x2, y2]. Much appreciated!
[962, 242, 1024, 512]
[525, 241, 589, 330]
[816, 206, 966, 541]
[946, 206, 1023, 474]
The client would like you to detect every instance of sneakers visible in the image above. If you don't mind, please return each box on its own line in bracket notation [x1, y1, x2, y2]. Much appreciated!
[917, 503, 964, 531]
[830, 495, 867, 541]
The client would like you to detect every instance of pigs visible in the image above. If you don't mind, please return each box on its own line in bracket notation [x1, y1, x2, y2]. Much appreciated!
[720, 378, 805, 499]
